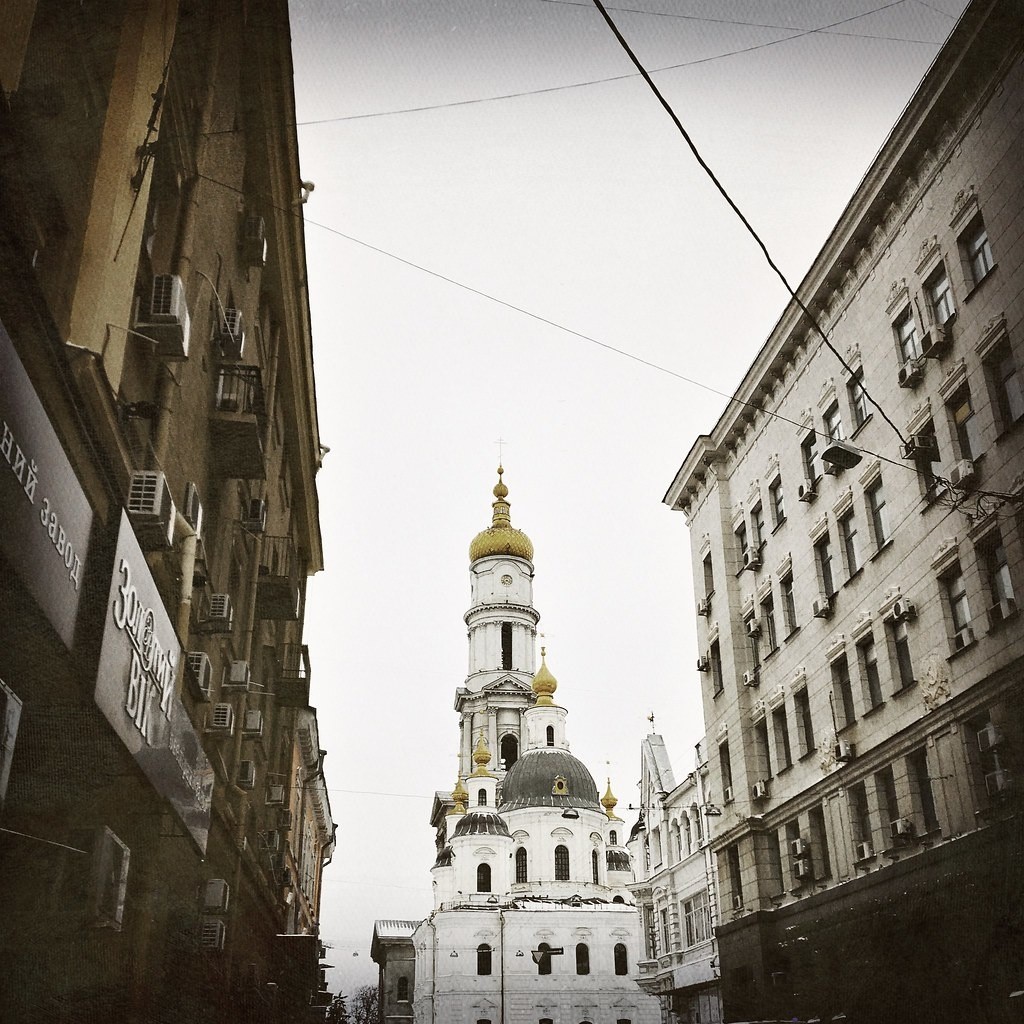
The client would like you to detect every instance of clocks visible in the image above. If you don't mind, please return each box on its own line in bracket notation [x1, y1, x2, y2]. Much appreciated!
[501, 575, 513, 585]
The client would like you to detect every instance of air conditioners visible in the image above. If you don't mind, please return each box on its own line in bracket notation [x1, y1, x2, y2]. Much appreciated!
[697, 599, 707, 616]
[976, 724, 1003, 753]
[888, 816, 910, 839]
[742, 547, 759, 569]
[896, 359, 922, 387]
[833, 739, 854, 763]
[792, 837, 810, 879]
[743, 670, 756, 685]
[857, 841, 873, 862]
[813, 596, 831, 618]
[697, 655, 708, 671]
[986, 769, 1013, 801]
[798, 477, 814, 501]
[920, 324, 946, 360]
[124, 210, 293, 953]
[891, 601, 909, 622]
[954, 598, 1017, 651]
[746, 618, 761, 639]
[945, 460, 979, 489]
[752, 782, 767, 802]
